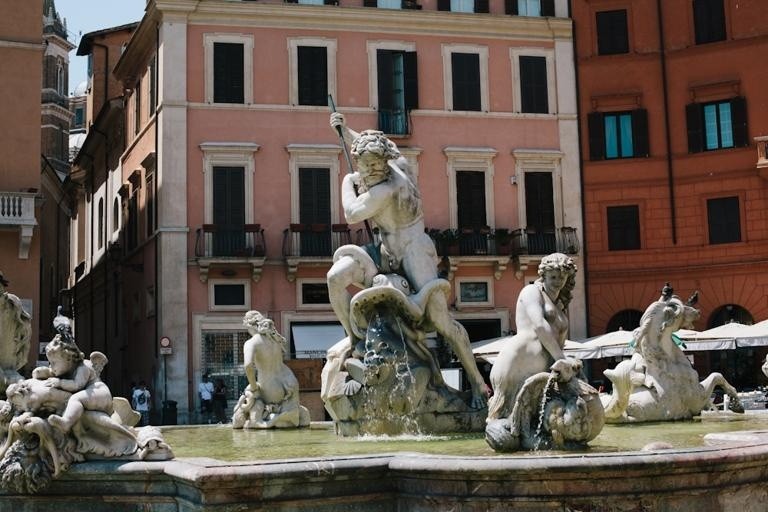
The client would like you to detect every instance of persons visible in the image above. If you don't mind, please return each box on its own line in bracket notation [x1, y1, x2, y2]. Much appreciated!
[31, 333, 116, 435]
[198, 374, 215, 424]
[326, 111, 492, 410]
[231, 310, 311, 430]
[213, 378, 228, 424]
[488, 252, 588, 419]
[131, 380, 151, 427]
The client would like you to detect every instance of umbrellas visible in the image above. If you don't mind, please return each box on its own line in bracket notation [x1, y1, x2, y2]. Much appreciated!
[450, 330, 602, 365]
[672, 328, 699, 339]
[580, 326, 634, 360]
[678, 318, 768, 351]
